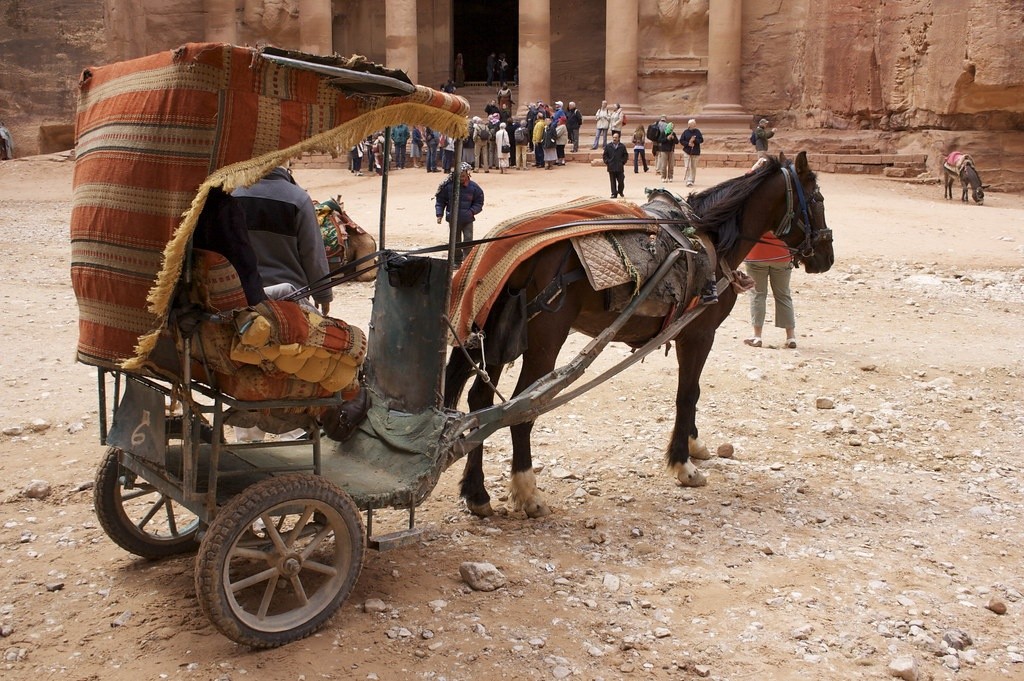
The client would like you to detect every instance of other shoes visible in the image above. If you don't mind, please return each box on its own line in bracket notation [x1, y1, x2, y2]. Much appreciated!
[610, 194, 617, 198]
[568, 140, 573, 144]
[432, 169, 441, 172]
[669, 178, 672, 182]
[785, 338, 795, 347]
[644, 167, 649, 172]
[746, 337, 763, 347]
[687, 181, 693, 187]
[470, 161, 566, 174]
[661, 178, 665, 182]
[618, 191, 624, 197]
[444, 169, 452, 173]
[427, 169, 433, 172]
[348, 162, 422, 176]
[453, 260, 462, 269]
[570, 149, 578, 152]
[590, 147, 597, 150]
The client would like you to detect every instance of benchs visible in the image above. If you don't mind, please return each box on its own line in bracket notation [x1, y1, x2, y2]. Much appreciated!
[174, 251, 365, 410]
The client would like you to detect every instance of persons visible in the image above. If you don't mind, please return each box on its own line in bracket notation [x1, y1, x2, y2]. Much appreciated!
[444, 80, 456, 94]
[755, 118, 777, 160]
[500, 103, 509, 122]
[462, 100, 568, 174]
[495, 54, 508, 87]
[435, 169, 484, 270]
[498, 82, 513, 116]
[653, 116, 667, 175]
[632, 125, 649, 173]
[347, 122, 457, 177]
[193, 183, 320, 314]
[229, 159, 333, 445]
[611, 103, 623, 138]
[603, 132, 628, 198]
[590, 100, 610, 150]
[485, 99, 499, 115]
[455, 53, 465, 88]
[657, 122, 679, 182]
[744, 157, 797, 350]
[565, 102, 582, 153]
[487, 53, 496, 87]
[680, 119, 704, 187]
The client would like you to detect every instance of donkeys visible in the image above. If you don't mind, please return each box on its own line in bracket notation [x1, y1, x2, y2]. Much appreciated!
[943, 152, 991, 206]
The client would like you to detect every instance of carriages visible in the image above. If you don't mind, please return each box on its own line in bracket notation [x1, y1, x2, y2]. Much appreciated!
[66, 40, 836, 653]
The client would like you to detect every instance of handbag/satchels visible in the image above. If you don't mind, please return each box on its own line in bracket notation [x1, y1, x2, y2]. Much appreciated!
[502, 146, 510, 153]
[356, 144, 363, 158]
[418, 141, 423, 147]
[647, 121, 660, 141]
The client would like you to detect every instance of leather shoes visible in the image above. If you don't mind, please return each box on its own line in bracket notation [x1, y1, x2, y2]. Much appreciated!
[322, 387, 370, 442]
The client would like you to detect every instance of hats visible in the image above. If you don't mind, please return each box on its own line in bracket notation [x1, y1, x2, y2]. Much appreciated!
[759, 118, 769, 124]
[555, 101, 563, 110]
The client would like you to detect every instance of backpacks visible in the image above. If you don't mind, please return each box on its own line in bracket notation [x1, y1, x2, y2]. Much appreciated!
[477, 124, 489, 140]
[750, 129, 760, 145]
[515, 128, 524, 142]
[618, 111, 626, 126]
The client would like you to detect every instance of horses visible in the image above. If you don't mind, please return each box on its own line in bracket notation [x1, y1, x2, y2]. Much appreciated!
[444, 151, 835, 519]
